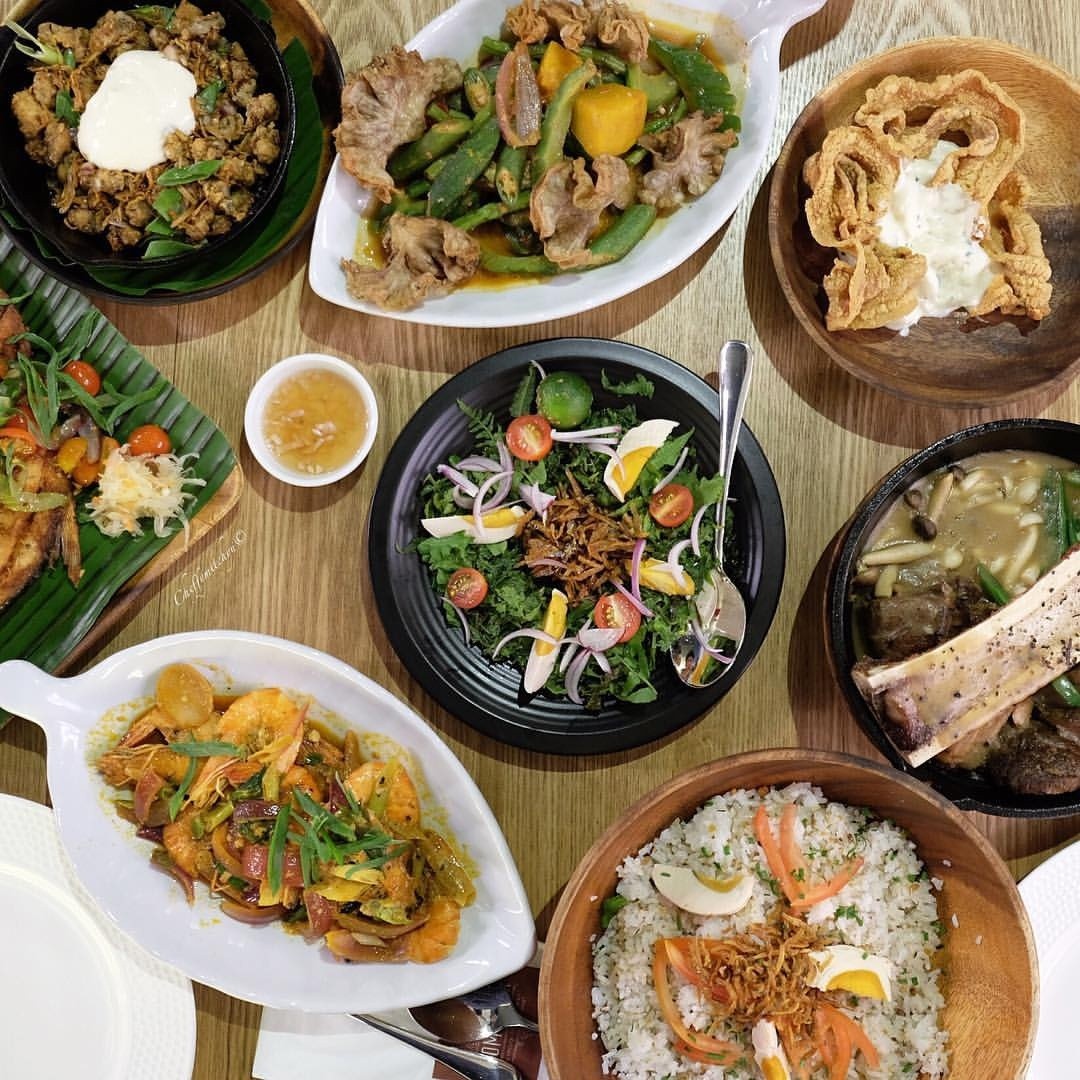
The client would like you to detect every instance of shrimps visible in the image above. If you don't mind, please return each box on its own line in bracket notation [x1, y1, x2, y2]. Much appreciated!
[349, 760, 426, 844]
[90, 701, 221, 788]
[223, 759, 323, 815]
[185, 687, 307, 816]
[162, 801, 216, 880]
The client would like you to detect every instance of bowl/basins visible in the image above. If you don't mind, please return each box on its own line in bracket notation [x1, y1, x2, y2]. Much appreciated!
[1, 0, 297, 275]
[365, 334, 786, 757]
[537, 741, 1039, 1079]
[826, 414, 1079, 822]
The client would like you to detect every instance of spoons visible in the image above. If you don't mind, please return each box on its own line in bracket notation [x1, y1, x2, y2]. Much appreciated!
[406, 982, 539, 1045]
[664, 337, 756, 689]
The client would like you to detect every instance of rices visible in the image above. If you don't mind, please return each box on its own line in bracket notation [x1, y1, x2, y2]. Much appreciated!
[587, 785, 952, 1080]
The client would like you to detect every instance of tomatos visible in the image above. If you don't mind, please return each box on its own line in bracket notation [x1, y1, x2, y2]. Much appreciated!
[0, 275, 170, 511]
[419, 371, 744, 702]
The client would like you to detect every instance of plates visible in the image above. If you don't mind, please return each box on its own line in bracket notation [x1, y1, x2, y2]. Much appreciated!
[767, 32, 1080, 408]
[240, 350, 378, 487]
[1, 629, 536, 1015]
[2, 227, 245, 731]
[1, 0, 354, 307]
[307, 1, 832, 326]
